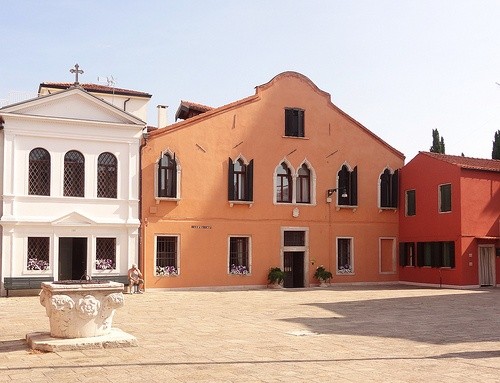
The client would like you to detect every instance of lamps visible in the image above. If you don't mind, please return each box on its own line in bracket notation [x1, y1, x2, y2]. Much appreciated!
[328, 186, 347, 198]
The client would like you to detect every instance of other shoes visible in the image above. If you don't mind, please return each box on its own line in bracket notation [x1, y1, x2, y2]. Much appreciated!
[138, 290, 143, 294]
[130, 291, 133, 294]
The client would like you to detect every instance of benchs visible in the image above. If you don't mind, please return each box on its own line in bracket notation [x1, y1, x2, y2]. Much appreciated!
[91, 275, 141, 293]
[3, 276, 55, 297]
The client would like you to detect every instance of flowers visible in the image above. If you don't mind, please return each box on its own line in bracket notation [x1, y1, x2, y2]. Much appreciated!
[27, 258, 49, 270]
[231, 264, 248, 275]
[155, 266, 177, 276]
[339, 264, 352, 274]
[96, 258, 114, 269]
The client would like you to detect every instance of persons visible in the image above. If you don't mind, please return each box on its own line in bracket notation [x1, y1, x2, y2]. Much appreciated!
[128, 264, 144, 295]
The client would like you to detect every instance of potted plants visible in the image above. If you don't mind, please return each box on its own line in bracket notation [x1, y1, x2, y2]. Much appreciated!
[312, 266, 332, 287]
[266, 266, 286, 288]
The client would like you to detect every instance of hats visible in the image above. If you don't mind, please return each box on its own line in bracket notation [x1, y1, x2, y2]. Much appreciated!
[133, 264, 137, 268]
